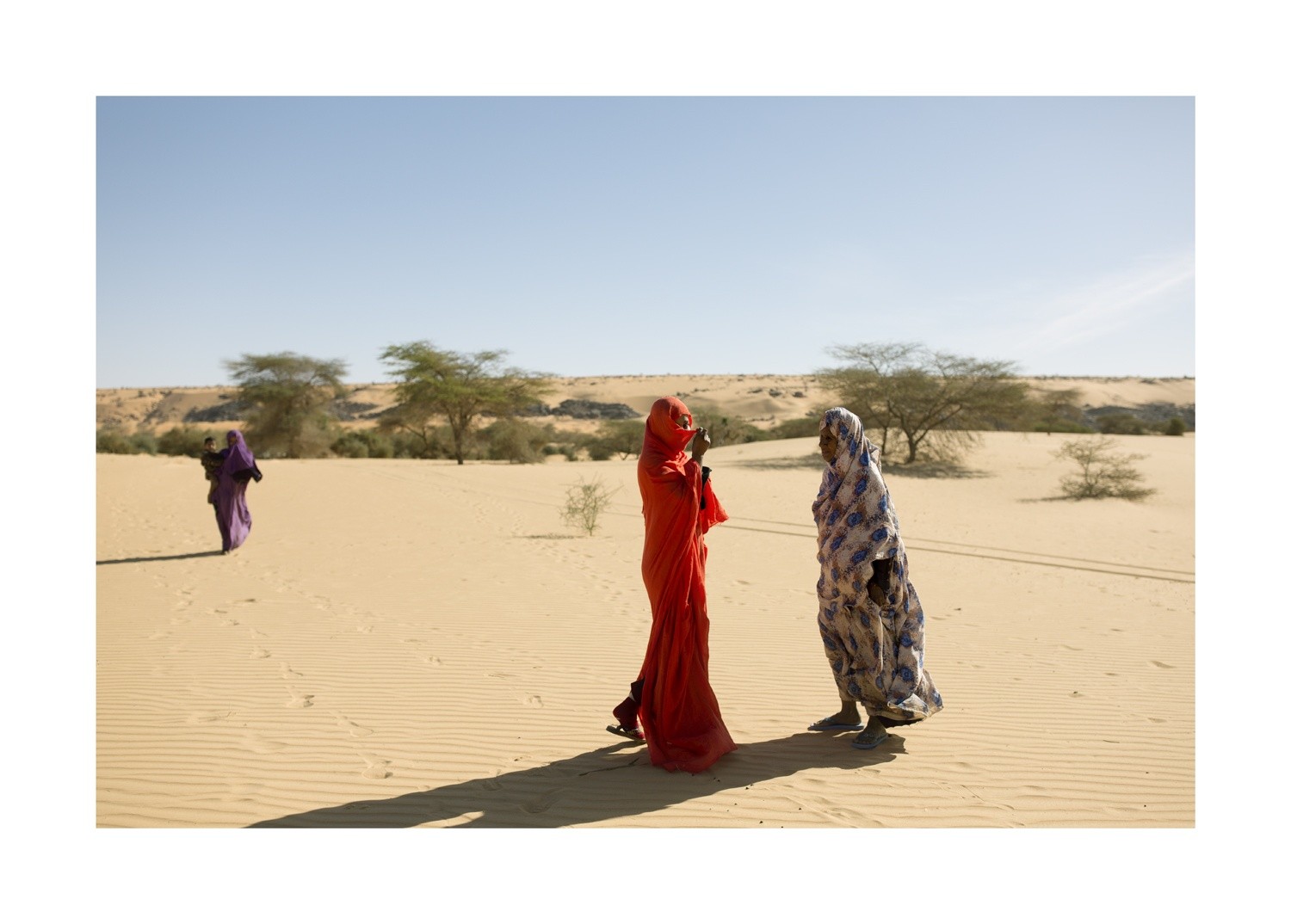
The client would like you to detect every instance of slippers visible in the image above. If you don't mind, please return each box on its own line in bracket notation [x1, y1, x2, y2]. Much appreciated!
[852, 732, 888, 749]
[809, 716, 864, 729]
[606, 724, 647, 742]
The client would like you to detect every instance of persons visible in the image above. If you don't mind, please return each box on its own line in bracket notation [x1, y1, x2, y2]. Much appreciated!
[606, 397, 738, 775]
[811, 407, 944, 750]
[202, 429, 262, 554]
